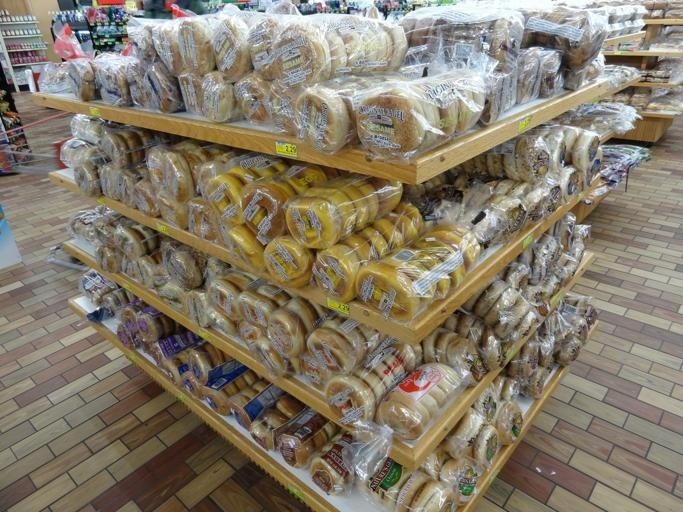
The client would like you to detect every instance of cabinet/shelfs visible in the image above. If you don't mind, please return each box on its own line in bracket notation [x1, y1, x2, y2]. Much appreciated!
[26, 63, 650, 512]
[1, 1, 156, 177]
[199, 1, 457, 21]
[598, 1, 683, 143]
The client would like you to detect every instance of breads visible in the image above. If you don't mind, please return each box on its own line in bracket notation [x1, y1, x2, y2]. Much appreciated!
[76, 266, 599, 512]
[65, 7, 609, 160]
[66, 200, 590, 441]
[58, 111, 602, 322]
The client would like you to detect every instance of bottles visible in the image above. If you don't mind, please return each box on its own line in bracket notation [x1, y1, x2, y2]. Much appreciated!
[0, 8, 47, 64]
[52, 9, 85, 23]
